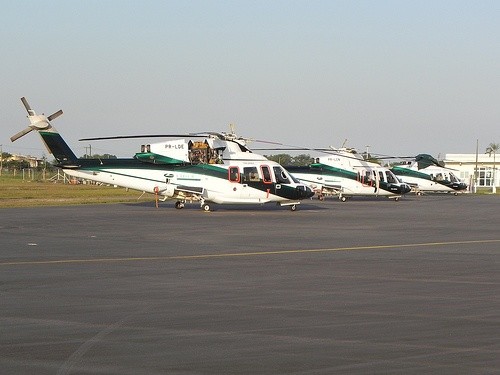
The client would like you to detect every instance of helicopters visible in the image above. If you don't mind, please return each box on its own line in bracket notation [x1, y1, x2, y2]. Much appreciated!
[10, 96, 468, 212]
